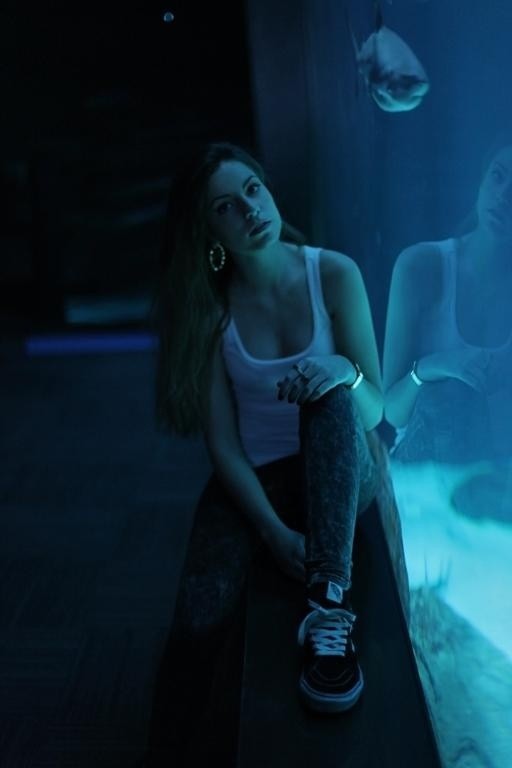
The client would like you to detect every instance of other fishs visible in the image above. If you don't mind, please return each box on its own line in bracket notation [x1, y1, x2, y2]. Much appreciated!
[347, 26, 430, 114]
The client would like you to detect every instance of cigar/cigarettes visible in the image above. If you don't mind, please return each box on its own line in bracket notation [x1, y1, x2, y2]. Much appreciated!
[290, 363, 309, 384]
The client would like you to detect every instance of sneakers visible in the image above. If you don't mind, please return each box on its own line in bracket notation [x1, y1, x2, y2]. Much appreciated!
[298, 589, 364, 712]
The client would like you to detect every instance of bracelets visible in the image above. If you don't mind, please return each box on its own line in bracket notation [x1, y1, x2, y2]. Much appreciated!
[410, 358, 425, 387]
[349, 360, 365, 392]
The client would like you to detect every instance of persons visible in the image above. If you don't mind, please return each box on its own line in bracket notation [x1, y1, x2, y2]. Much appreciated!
[146, 142, 388, 768]
[383, 143, 512, 768]
